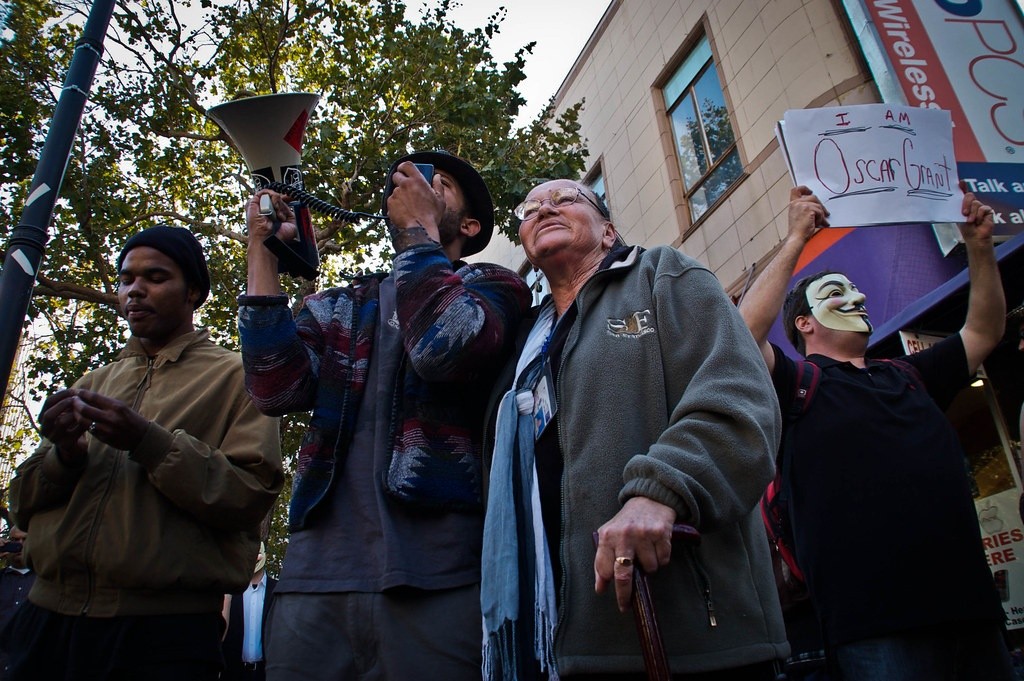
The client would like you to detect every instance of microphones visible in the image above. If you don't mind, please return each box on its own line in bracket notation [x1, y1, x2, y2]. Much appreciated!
[415, 164, 434, 187]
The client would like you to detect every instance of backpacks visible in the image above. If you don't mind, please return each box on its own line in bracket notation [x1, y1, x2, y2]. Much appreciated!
[759, 474, 808, 584]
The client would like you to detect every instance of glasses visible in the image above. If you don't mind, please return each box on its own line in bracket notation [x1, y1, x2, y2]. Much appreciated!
[513, 187, 611, 221]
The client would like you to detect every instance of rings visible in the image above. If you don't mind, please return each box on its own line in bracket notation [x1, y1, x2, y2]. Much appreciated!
[87, 421, 98, 436]
[615, 557, 633, 567]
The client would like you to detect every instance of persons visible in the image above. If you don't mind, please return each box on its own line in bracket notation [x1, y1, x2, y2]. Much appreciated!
[477, 177, 791, 679]
[5, 226, 285, 681]
[1, 525, 37, 635]
[239, 149, 536, 681]
[738, 183, 1017, 681]
[243, 539, 284, 681]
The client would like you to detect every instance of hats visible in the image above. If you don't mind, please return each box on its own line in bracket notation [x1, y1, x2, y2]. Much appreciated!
[382, 149, 494, 258]
[118, 226, 211, 309]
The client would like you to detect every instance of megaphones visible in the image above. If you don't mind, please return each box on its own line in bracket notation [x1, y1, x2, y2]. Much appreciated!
[204, 92, 319, 282]
